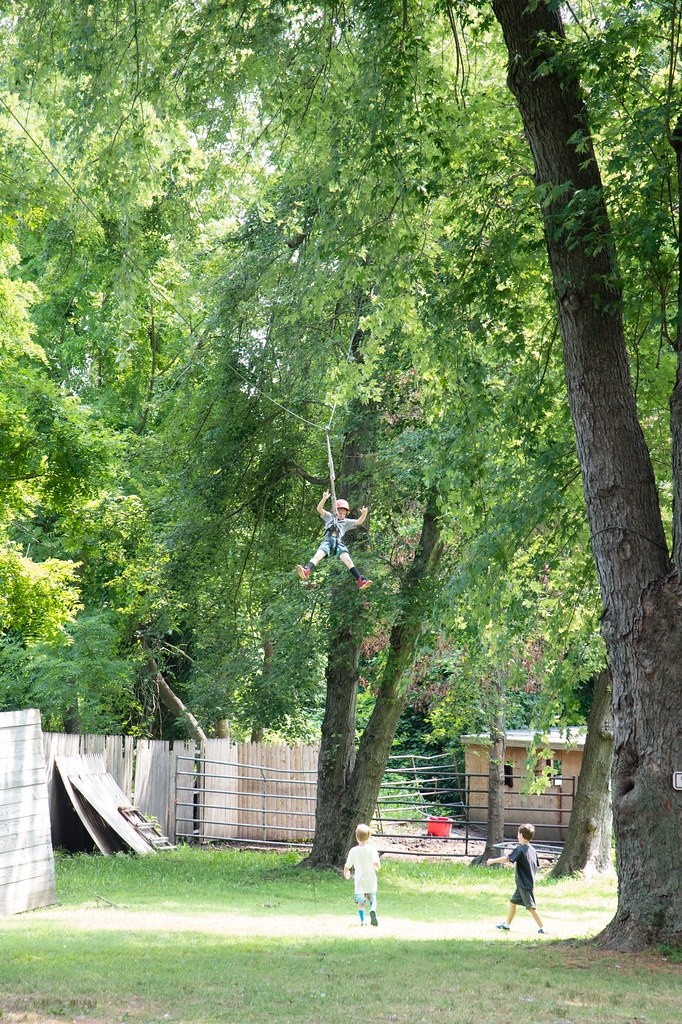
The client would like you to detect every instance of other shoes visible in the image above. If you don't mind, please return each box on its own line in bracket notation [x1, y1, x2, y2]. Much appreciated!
[369, 909, 379, 927]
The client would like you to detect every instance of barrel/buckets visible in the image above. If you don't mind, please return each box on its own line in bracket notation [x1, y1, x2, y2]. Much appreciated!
[427, 816, 453, 842]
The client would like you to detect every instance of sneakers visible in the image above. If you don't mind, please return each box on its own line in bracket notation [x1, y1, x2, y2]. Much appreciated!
[495, 922, 510, 931]
[538, 929, 549, 934]
[357, 575, 373, 590]
[297, 566, 311, 580]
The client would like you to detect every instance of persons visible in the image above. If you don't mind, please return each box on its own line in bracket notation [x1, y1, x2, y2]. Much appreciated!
[296, 488, 376, 592]
[342, 824, 382, 927]
[486, 822, 546, 934]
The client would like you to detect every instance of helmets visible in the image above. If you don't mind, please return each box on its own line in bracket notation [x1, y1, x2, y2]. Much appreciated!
[331, 499, 350, 512]
